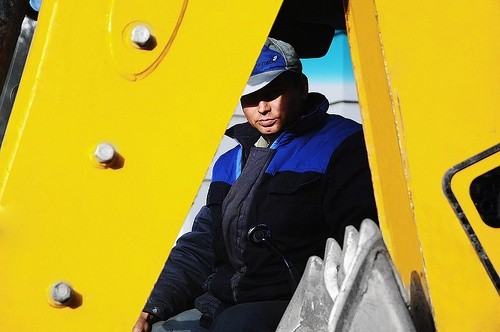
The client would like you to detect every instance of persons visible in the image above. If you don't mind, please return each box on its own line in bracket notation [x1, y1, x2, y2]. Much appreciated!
[133, 38, 375, 332]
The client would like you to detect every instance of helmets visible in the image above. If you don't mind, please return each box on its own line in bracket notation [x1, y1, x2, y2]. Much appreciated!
[241, 37, 303, 97]
